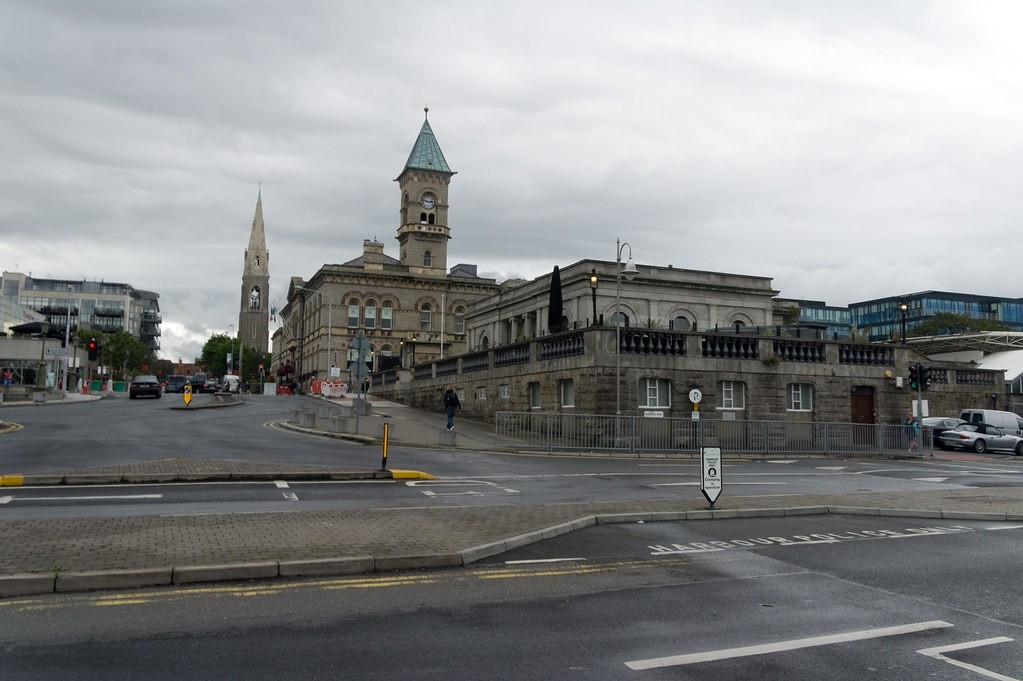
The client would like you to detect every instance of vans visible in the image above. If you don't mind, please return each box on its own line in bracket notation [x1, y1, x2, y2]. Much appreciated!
[165, 375, 188, 393]
[959, 409, 1023, 436]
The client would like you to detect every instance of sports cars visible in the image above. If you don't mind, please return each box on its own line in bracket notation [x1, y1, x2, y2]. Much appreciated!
[939, 422, 1023, 455]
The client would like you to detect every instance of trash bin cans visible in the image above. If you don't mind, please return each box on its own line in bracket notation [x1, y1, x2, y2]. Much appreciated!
[92, 380, 102, 391]
[113, 382, 125, 392]
[33, 387, 47, 403]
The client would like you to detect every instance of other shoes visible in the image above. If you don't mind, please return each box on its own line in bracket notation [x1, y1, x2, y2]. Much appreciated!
[450, 426, 454, 430]
[446, 427, 449, 431]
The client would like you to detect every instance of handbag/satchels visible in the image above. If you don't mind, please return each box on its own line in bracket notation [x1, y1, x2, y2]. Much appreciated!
[444, 393, 449, 411]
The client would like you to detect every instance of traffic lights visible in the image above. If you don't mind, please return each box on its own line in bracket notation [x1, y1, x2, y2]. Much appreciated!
[920, 364, 933, 392]
[908, 363, 919, 392]
[87, 340, 97, 361]
[259, 364, 263, 374]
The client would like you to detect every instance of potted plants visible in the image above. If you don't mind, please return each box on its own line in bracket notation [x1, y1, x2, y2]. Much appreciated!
[91, 375, 103, 391]
[113, 372, 126, 392]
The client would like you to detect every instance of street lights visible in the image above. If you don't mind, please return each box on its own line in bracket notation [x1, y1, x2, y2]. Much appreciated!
[293, 285, 331, 383]
[612, 238, 641, 447]
[105, 343, 116, 394]
[400, 337, 404, 369]
[30, 316, 51, 402]
[588, 267, 601, 326]
[411, 334, 416, 365]
[229, 324, 234, 375]
[59, 286, 74, 398]
[898, 301, 909, 344]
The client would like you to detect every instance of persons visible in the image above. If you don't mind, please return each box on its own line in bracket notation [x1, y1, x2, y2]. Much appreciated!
[444, 385, 461, 431]
[905, 412, 919, 451]
[0, 367, 12, 385]
[59, 377, 62, 390]
[362, 377, 370, 394]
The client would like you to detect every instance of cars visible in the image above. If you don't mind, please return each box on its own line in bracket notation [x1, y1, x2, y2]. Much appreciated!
[907, 417, 972, 448]
[129, 375, 162, 397]
[189, 373, 221, 393]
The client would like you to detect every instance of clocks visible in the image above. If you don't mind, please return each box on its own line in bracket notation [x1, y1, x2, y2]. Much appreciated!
[421, 194, 435, 210]
